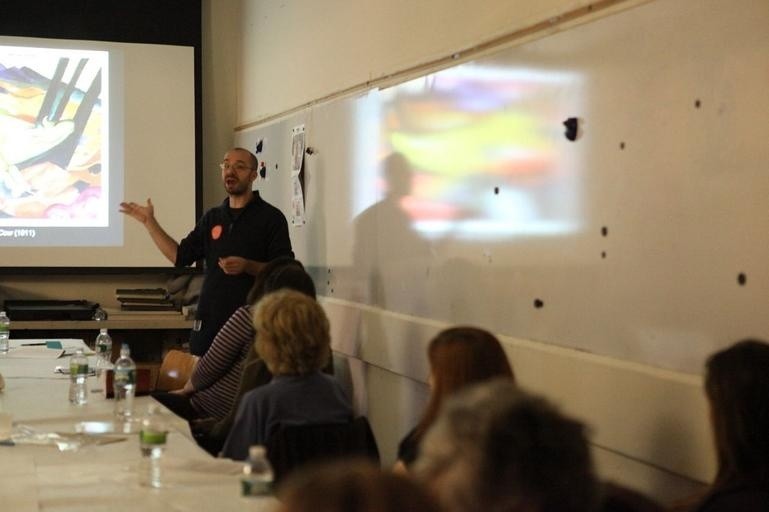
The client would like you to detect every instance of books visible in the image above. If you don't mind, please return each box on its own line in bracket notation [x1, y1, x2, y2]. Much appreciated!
[116, 289, 175, 311]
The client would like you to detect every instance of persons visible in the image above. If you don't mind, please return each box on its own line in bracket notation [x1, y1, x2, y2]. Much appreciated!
[185, 265, 318, 458]
[118, 148, 295, 354]
[218, 287, 352, 459]
[153, 256, 335, 422]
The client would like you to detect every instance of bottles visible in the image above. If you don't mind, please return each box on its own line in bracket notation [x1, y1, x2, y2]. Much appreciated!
[239, 445, 275, 512]
[139, 404, 169, 477]
[1, 309, 10, 356]
[93, 328, 113, 370]
[112, 343, 137, 414]
[67, 346, 89, 406]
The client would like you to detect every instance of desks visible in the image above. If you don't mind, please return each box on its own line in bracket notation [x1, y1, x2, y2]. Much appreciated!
[0, 333, 282, 512]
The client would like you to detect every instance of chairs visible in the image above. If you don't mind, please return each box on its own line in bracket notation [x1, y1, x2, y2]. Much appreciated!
[258, 414, 382, 485]
[154, 351, 202, 390]
[194, 358, 349, 455]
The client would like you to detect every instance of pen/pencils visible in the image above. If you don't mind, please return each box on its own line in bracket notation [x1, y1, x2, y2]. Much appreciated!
[21, 343, 46, 346]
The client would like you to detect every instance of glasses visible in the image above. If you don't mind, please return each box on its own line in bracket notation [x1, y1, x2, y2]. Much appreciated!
[219, 161, 255, 172]
